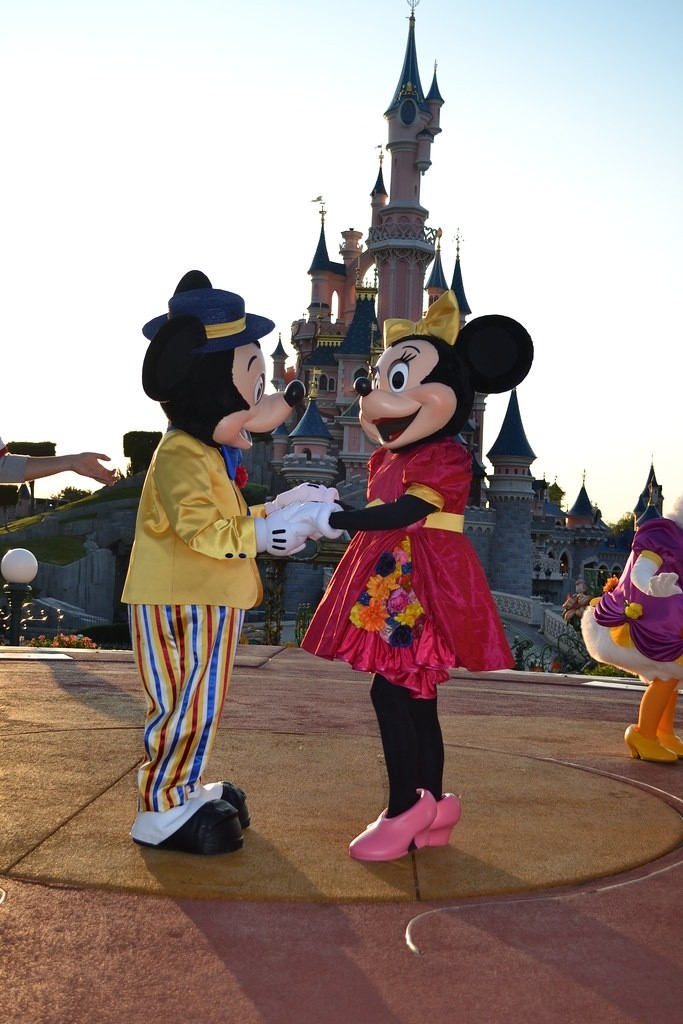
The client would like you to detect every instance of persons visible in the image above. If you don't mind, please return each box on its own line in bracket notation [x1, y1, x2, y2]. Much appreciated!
[0, 438, 119, 486]
[576, 580, 588, 597]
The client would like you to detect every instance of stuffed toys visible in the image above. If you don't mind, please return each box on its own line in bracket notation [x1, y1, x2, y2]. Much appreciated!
[119, 270, 312, 854]
[579, 495, 683, 760]
[308, 290, 533, 861]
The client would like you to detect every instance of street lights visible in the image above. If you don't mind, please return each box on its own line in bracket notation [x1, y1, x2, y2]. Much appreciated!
[1, 548, 38, 648]
